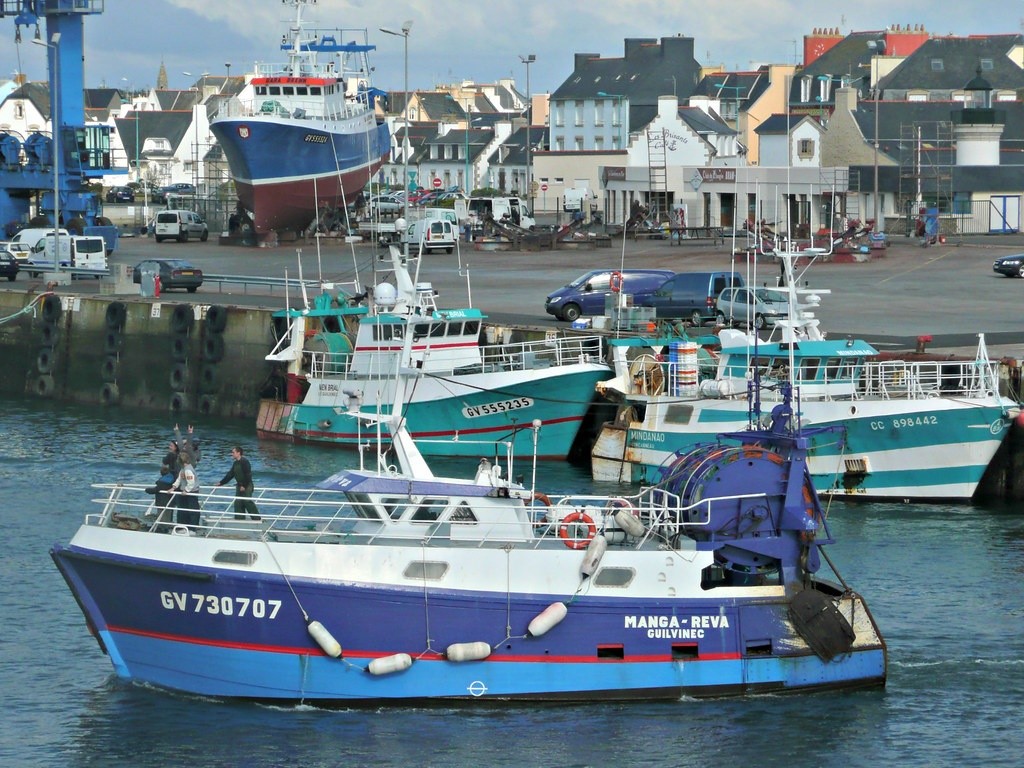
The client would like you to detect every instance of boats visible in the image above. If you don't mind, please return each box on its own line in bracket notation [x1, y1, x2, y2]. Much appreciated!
[208, 0, 391, 235]
[591, 72, 1024, 507]
[49, 177, 889, 710]
[254, 121, 614, 462]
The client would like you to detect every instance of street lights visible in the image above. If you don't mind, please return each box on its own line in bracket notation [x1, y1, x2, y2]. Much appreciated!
[381, 20, 415, 264]
[521, 53, 536, 216]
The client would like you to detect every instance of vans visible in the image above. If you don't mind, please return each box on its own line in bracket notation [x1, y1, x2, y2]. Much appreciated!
[642, 271, 746, 327]
[545, 269, 674, 322]
[12, 228, 109, 280]
[407, 218, 456, 254]
[453, 196, 537, 238]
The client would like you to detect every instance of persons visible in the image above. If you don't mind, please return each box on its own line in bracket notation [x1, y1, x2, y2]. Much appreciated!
[213, 447, 261, 520]
[145, 423, 201, 537]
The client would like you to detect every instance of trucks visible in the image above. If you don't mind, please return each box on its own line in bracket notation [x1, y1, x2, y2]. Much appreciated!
[358, 207, 460, 247]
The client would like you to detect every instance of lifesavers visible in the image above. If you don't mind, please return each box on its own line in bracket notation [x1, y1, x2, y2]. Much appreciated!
[201, 333, 224, 363]
[98, 384, 125, 404]
[198, 365, 221, 393]
[196, 396, 219, 416]
[169, 392, 189, 413]
[104, 332, 124, 353]
[106, 302, 126, 328]
[205, 305, 227, 332]
[170, 364, 192, 390]
[170, 334, 192, 359]
[35, 375, 54, 397]
[40, 325, 61, 346]
[100, 356, 121, 382]
[172, 304, 195, 332]
[523, 492, 551, 528]
[610, 272, 621, 292]
[43, 296, 61, 322]
[36, 348, 56, 373]
[560, 513, 596, 549]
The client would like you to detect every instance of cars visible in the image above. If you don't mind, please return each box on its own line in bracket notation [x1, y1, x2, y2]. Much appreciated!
[716, 287, 789, 330]
[152, 183, 196, 204]
[992, 254, 1024, 279]
[133, 258, 203, 293]
[105, 186, 135, 203]
[0, 251, 19, 282]
[155, 210, 209, 243]
[0, 242, 31, 263]
[347, 187, 470, 215]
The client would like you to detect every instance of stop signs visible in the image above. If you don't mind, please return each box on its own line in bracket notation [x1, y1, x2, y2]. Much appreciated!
[433, 179, 442, 187]
